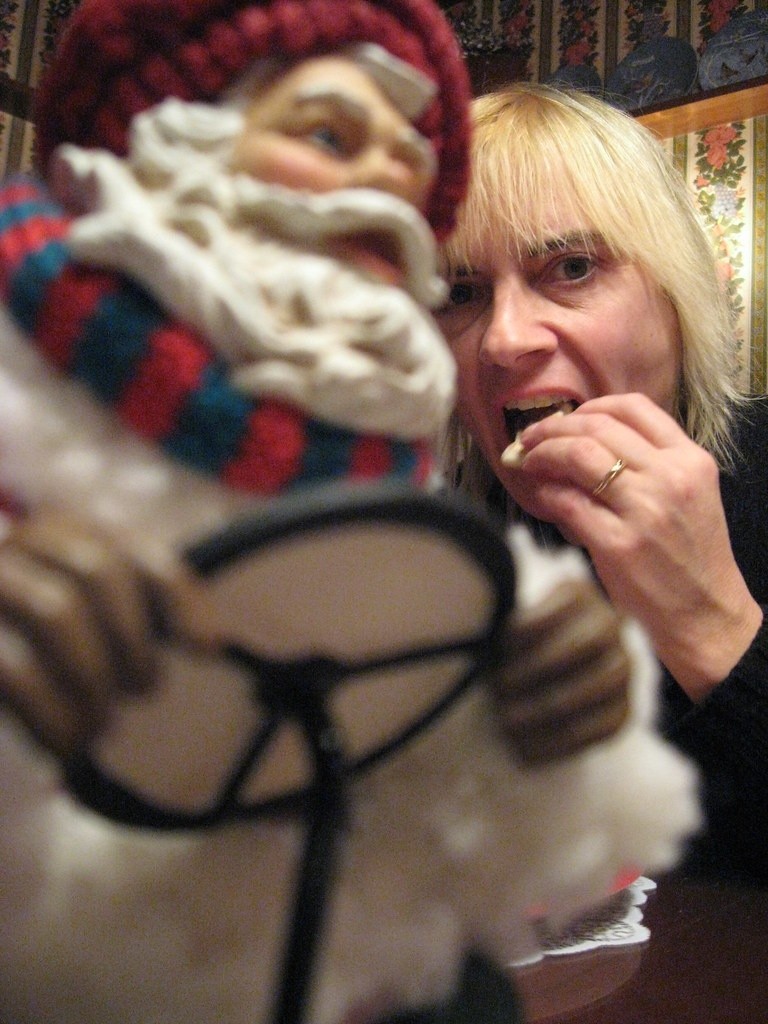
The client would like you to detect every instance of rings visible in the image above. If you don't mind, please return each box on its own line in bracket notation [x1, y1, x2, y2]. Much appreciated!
[593, 459, 625, 497]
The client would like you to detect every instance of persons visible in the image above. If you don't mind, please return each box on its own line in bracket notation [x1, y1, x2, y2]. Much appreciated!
[434, 88, 768, 882]
[1, 0, 697, 1024]
